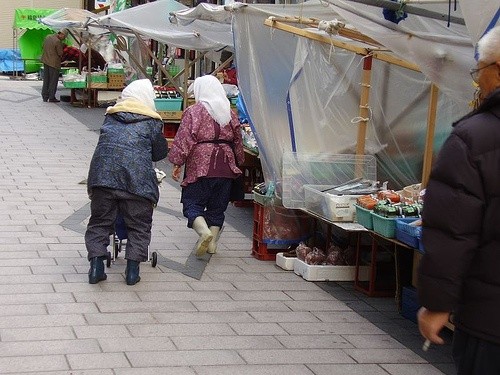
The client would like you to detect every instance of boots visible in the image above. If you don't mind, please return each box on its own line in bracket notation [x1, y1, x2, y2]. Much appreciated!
[88, 258, 107, 284]
[207, 225, 220, 254]
[125, 259, 140, 285]
[192, 216, 213, 257]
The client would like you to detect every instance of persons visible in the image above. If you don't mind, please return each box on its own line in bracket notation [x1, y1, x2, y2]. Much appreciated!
[40, 28, 68, 102]
[80, 44, 108, 99]
[170, 75, 245, 257]
[60, 43, 87, 101]
[83, 78, 168, 284]
[418, 24, 500, 375]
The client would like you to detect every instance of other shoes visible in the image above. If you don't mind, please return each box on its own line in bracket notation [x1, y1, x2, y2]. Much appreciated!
[49, 97, 60, 102]
[43, 99, 47, 102]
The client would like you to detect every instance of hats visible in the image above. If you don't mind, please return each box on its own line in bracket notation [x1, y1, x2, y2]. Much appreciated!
[59, 28, 68, 36]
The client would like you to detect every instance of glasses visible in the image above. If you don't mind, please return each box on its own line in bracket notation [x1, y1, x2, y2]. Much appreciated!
[470, 61, 497, 84]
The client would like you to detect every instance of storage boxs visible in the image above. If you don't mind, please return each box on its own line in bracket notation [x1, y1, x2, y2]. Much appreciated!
[154, 96, 183, 111]
[233, 160, 424, 321]
[63, 69, 124, 88]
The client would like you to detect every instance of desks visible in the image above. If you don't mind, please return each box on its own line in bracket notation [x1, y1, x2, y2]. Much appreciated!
[301, 207, 413, 297]
[71, 87, 123, 108]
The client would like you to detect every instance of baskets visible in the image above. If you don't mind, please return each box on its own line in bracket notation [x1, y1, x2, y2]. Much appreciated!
[252, 190, 266, 205]
[417, 227, 423, 254]
[352, 203, 373, 230]
[370, 209, 398, 239]
[394, 218, 423, 246]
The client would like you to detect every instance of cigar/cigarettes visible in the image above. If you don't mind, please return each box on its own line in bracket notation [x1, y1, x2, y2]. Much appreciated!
[422, 334, 430, 351]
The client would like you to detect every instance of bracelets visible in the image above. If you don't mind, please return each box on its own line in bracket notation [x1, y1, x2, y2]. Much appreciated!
[175, 165, 179, 167]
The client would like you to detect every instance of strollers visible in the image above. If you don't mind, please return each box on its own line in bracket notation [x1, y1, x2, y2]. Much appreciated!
[104, 169, 168, 268]
